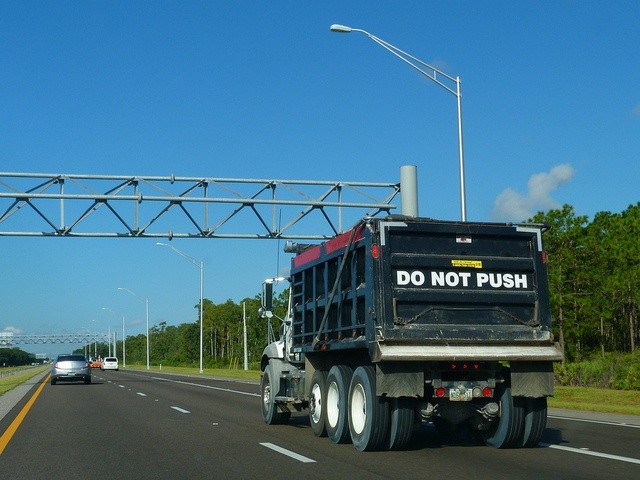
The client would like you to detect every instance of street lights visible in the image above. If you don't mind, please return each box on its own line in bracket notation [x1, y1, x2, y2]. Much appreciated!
[331, 23, 468, 222]
[118, 287, 150, 372]
[156, 242, 206, 375]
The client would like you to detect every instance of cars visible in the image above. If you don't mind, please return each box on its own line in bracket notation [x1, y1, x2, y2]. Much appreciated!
[51, 353, 91, 385]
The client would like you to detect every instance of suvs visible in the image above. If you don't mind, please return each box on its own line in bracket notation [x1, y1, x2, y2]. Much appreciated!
[100, 356, 119, 372]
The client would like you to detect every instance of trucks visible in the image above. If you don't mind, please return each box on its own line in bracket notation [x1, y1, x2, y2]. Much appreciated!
[260, 213, 564, 451]
